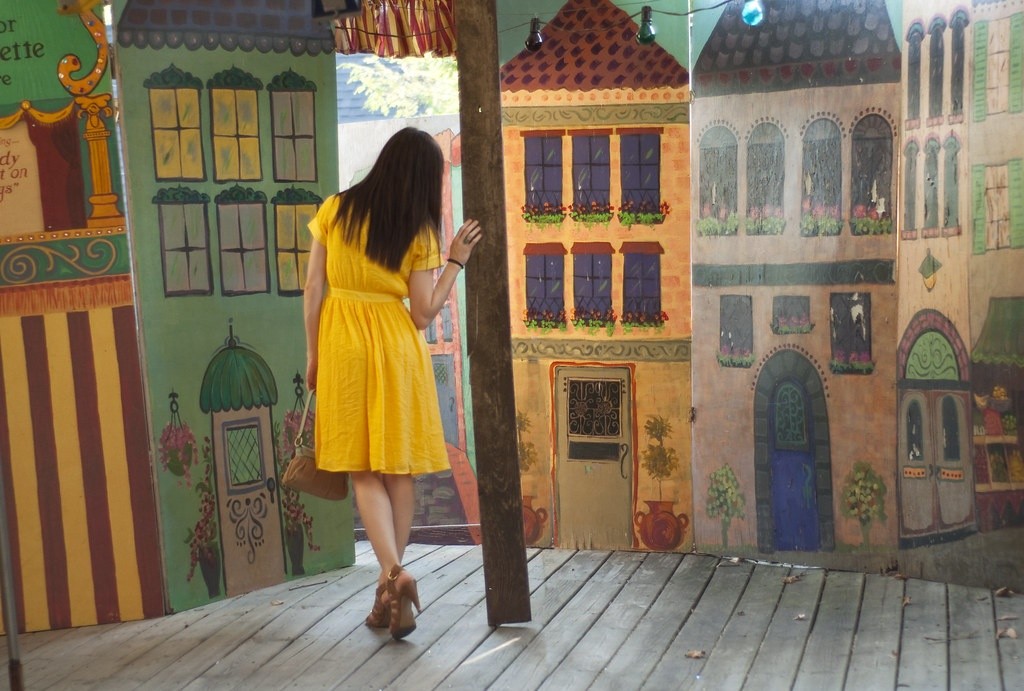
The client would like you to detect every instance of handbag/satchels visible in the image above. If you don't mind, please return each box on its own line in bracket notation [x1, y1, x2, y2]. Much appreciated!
[281, 388, 349, 500]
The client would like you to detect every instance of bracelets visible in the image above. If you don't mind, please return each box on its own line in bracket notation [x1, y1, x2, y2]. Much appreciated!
[446, 258, 464, 269]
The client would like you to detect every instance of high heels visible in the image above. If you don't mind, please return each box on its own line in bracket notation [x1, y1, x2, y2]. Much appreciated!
[387, 565, 421, 640]
[366, 580, 390, 628]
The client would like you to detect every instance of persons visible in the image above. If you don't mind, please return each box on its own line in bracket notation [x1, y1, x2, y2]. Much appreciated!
[304, 128, 482, 644]
